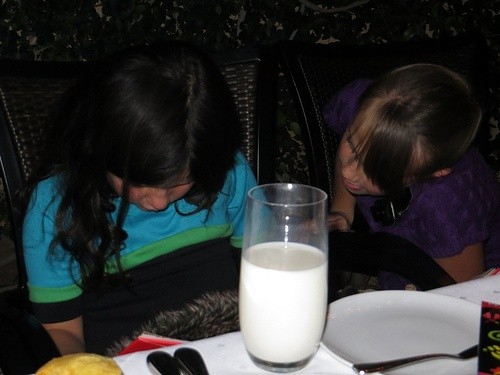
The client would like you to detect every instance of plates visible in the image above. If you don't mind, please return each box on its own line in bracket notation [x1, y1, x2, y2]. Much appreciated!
[320, 289, 480, 375]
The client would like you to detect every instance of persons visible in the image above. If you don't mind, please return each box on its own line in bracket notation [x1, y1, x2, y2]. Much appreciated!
[296, 64, 499, 286]
[20, 39, 272, 355]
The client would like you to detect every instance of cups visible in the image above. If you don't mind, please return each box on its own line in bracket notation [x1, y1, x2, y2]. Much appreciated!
[238, 182, 328, 373]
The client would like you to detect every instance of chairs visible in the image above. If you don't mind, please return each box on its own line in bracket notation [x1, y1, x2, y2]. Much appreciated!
[0, 31, 492, 375]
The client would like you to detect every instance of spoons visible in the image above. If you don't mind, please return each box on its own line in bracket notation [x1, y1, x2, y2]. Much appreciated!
[351, 345, 479, 375]
